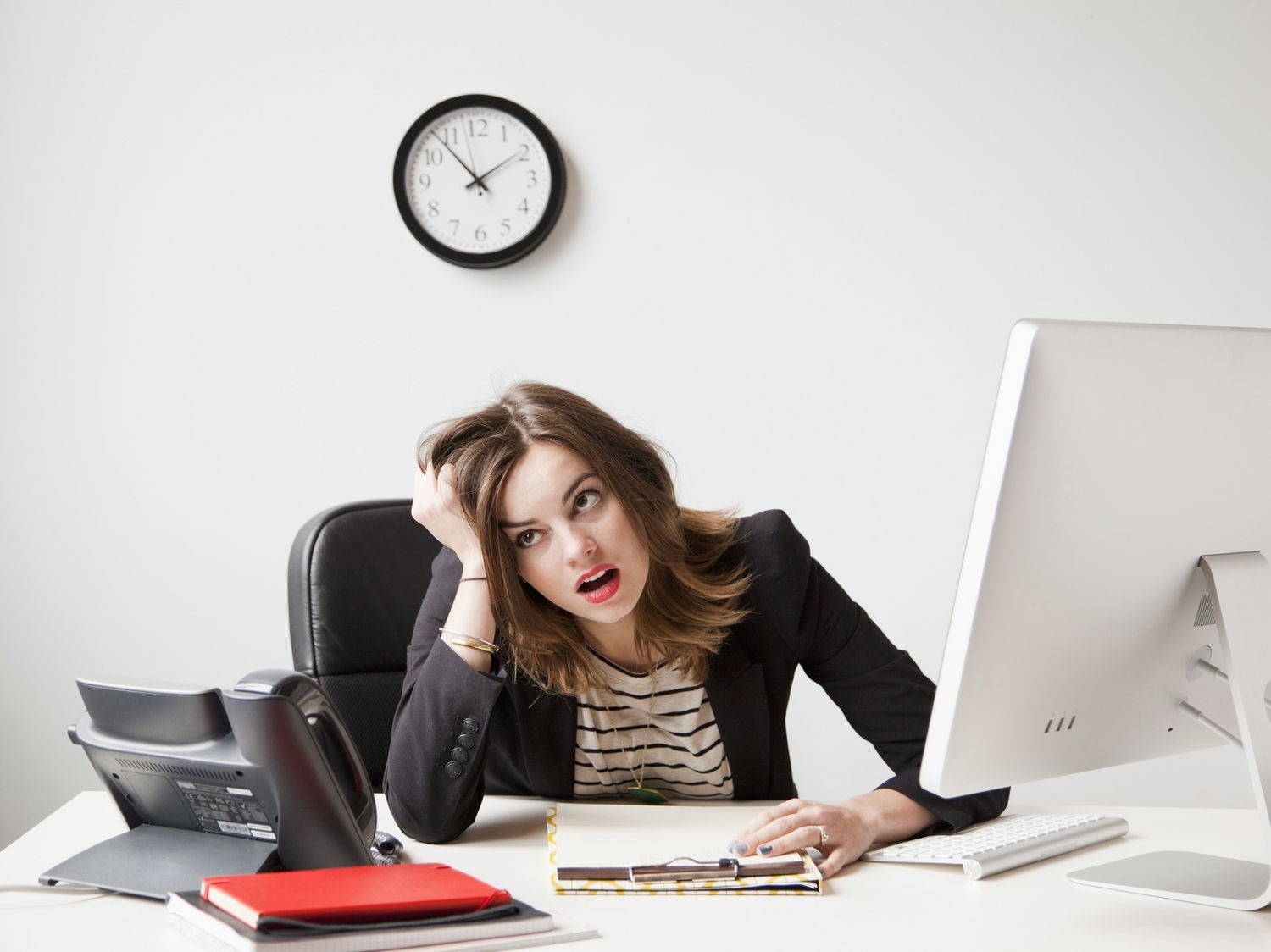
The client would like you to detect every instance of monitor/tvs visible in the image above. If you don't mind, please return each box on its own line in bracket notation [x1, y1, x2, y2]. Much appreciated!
[918, 316, 1271, 911]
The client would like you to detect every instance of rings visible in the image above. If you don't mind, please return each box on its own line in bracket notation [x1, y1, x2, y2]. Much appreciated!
[814, 825, 829, 847]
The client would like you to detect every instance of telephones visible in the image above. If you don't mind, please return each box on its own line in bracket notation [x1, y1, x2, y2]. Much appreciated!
[39, 672, 378, 901]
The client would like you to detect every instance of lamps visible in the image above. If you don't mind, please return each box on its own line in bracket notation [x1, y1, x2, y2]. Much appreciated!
[392, 93, 568, 270]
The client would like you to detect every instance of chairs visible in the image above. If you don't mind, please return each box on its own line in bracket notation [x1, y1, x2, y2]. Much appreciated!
[288, 500, 443, 793]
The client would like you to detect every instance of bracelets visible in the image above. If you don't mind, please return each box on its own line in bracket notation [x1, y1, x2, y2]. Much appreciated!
[460, 569, 487, 581]
[439, 627, 499, 655]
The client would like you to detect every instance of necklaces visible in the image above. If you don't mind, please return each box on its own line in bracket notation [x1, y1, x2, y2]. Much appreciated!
[587, 651, 668, 802]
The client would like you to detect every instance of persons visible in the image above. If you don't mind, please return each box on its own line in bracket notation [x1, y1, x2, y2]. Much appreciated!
[382, 383, 1010, 881]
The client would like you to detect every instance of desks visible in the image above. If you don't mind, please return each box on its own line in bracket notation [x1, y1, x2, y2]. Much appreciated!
[1, 787, 1270, 952]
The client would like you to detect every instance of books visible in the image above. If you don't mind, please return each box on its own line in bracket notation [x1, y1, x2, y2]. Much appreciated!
[166, 863, 602, 952]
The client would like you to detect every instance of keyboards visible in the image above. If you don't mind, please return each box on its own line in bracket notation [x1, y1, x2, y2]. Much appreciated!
[861, 814, 1129, 882]
